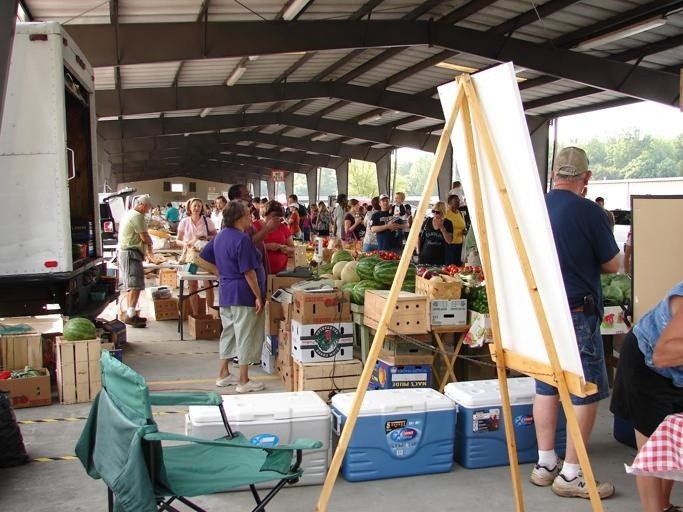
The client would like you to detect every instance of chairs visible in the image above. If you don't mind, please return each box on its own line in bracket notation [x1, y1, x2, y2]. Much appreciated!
[73, 345, 324, 512]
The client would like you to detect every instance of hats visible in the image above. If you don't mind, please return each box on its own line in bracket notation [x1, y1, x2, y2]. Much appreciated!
[554, 148, 594, 181]
[289, 203, 300, 210]
[376, 192, 391, 203]
[137, 194, 159, 211]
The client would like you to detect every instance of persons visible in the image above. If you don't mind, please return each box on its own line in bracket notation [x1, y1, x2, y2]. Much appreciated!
[610, 282, 683, 512]
[623, 229, 633, 273]
[530, 146, 623, 499]
[595, 197, 615, 233]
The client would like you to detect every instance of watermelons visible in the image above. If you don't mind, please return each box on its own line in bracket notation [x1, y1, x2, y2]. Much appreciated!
[372, 259, 417, 285]
[356, 257, 380, 279]
[402, 279, 416, 293]
[353, 280, 386, 306]
[342, 282, 360, 301]
[20, 369, 44, 395]
[61, 317, 98, 341]
[331, 250, 353, 267]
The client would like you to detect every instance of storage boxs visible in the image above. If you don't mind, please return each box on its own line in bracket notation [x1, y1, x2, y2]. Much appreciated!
[185, 390, 333, 492]
[332, 388, 456, 482]
[600, 300, 631, 335]
[613, 415, 636, 449]
[2, 313, 126, 410]
[261, 274, 364, 402]
[443, 377, 567, 469]
[364, 274, 494, 391]
[143, 246, 222, 340]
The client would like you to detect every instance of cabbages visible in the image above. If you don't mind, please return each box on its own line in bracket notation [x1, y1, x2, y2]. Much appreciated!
[601, 273, 631, 306]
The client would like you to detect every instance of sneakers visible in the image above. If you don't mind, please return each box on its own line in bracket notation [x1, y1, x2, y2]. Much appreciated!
[118, 309, 151, 328]
[553, 471, 618, 501]
[235, 380, 264, 394]
[215, 374, 241, 388]
[529, 461, 570, 488]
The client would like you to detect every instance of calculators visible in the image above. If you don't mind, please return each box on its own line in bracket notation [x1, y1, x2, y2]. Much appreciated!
[271, 289, 292, 302]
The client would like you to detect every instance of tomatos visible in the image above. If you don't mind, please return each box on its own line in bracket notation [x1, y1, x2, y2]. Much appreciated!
[365, 249, 400, 259]
[442, 264, 484, 282]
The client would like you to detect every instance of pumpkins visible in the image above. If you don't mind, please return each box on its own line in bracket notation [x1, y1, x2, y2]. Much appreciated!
[320, 260, 360, 288]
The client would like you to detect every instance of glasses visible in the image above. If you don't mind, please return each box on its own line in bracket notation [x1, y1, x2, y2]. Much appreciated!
[432, 210, 442, 214]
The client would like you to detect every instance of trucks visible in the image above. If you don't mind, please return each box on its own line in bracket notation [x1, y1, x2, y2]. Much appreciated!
[95, 187, 139, 265]
[0, 22, 122, 319]
